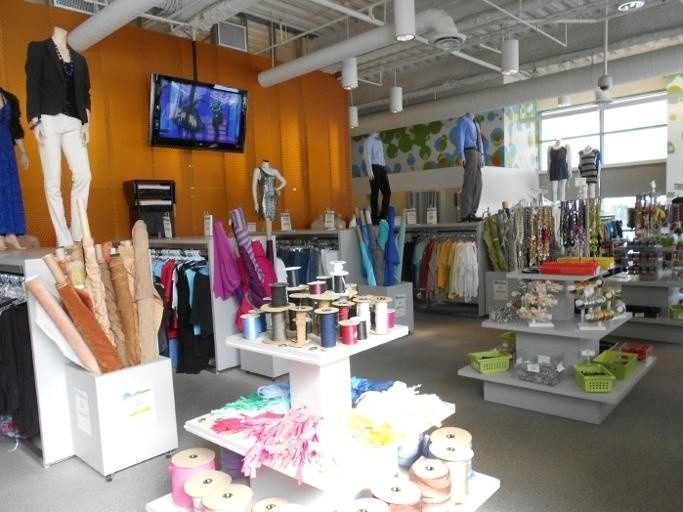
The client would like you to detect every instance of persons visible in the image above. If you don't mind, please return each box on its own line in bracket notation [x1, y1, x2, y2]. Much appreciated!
[363, 131, 391, 225]
[454, 111, 484, 222]
[577, 145, 602, 199]
[25, 27, 91, 251]
[251, 160, 287, 240]
[0, 89, 31, 249]
[546, 139, 571, 203]
[212, 112, 223, 141]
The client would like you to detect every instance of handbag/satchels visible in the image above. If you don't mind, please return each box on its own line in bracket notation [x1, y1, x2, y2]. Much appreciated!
[627, 204, 683, 233]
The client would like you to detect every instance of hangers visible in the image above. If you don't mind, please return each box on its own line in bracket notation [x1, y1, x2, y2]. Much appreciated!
[274, 238, 339, 258]
[147, 247, 208, 272]
[0, 274, 26, 309]
[408, 231, 477, 245]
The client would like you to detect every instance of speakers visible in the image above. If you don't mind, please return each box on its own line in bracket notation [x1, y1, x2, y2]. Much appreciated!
[212, 20, 247, 52]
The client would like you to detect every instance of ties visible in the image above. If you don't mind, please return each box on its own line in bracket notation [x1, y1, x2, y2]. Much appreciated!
[473, 119, 478, 145]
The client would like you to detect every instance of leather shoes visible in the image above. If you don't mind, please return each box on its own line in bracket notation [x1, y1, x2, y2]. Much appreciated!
[460, 213, 483, 222]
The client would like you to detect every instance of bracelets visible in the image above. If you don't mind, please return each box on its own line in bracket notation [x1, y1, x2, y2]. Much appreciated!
[574, 276, 628, 324]
[29, 120, 41, 130]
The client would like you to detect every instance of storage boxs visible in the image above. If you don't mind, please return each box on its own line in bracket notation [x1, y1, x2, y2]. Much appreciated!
[65, 353, 179, 483]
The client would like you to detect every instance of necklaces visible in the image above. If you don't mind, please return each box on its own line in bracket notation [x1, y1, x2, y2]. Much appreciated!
[262, 166, 271, 174]
[50, 38, 73, 76]
[584, 148, 591, 152]
[0, 91, 5, 123]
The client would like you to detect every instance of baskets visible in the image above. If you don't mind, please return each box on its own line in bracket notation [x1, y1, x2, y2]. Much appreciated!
[573, 364, 616, 393]
[467, 350, 512, 374]
[501, 332, 516, 349]
[592, 350, 637, 381]
[669, 305, 683, 320]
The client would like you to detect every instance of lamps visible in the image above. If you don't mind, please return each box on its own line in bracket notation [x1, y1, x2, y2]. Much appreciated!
[393, 0, 416, 42]
[348, 90, 358, 130]
[341, 57, 358, 91]
[501, 23, 519, 74]
[389, 67, 403, 113]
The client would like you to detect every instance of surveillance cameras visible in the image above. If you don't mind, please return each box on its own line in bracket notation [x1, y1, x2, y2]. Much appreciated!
[598, 75, 612, 91]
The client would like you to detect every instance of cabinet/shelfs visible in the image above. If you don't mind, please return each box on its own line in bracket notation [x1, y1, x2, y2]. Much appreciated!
[146, 321, 500, 512]
[605, 244, 683, 345]
[458, 267, 656, 425]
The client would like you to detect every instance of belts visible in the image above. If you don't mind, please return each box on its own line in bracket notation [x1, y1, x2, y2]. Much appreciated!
[465, 148, 478, 151]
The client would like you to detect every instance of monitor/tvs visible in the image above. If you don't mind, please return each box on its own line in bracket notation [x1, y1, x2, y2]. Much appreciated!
[149, 72, 248, 153]
